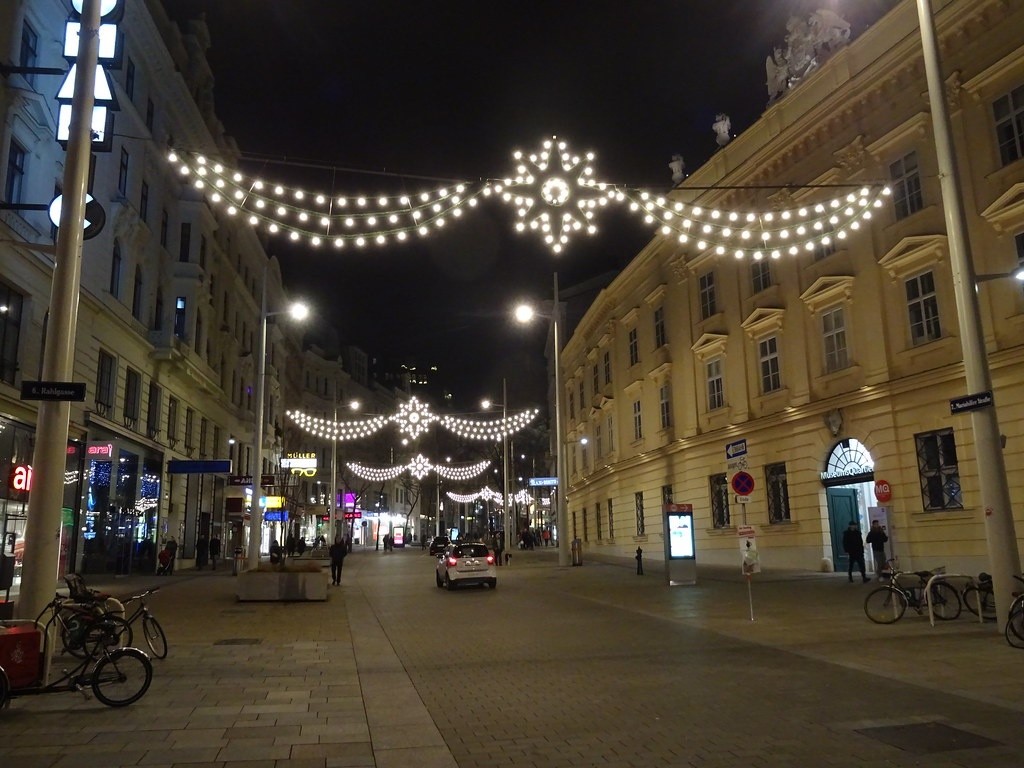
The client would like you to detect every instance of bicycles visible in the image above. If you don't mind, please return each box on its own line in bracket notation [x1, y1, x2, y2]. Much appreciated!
[864, 558, 962, 624]
[0, 572, 169, 708]
[962, 572, 997, 621]
[1005, 575, 1024, 649]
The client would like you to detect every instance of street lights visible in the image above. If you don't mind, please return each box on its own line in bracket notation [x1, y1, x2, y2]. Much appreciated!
[246, 302, 309, 565]
[516, 273, 573, 567]
[481, 377, 511, 552]
[329, 392, 359, 547]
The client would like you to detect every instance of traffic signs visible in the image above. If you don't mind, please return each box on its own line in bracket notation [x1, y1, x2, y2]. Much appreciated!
[726, 438, 748, 459]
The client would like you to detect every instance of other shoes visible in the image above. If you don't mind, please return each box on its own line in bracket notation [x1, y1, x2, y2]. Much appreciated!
[332, 580, 336, 585]
[863, 578, 871, 584]
[337, 581, 340, 586]
[877, 576, 885, 583]
[848, 576, 853, 584]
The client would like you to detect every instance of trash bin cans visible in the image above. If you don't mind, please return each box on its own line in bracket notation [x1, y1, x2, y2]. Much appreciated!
[233, 545, 244, 576]
[354, 539, 359, 544]
[572, 538, 582, 566]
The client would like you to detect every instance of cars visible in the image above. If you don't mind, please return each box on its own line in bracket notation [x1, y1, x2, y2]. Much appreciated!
[436, 541, 497, 589]
[430, 536, 452, 556]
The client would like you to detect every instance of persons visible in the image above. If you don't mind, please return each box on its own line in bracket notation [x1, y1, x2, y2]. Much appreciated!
[195, 533, 208, 570]
[383, 534, 394, 551]
[510, 524, 550, 550]
[270, 540, 282, 564]
[329, 535, 346, 586]
[493, 533, 504, 566]
[421, 534, 428, 550]
[210, 533, 220, 570]
[285, 534, 306, 556]
[843, 521, 871, 583]
[866, 520, 889, 577]
[315, 536, 320, 550]
[164, 536, 177, 575]
[408, 531, 412, 543]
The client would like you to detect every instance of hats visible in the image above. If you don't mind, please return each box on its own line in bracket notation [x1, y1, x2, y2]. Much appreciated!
[848, 520, 858, 526]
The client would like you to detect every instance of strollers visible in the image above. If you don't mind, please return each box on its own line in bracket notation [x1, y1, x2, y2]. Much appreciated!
[156, 549, 173, 577]
